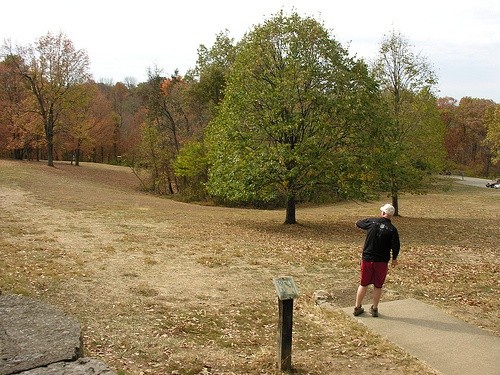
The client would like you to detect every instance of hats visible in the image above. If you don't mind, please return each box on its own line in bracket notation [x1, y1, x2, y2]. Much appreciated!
[380, 203, 395, 216]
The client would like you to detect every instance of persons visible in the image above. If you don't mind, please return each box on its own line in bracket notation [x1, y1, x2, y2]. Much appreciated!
[484, 180, 499, 188]
[353, 204, 401, 318]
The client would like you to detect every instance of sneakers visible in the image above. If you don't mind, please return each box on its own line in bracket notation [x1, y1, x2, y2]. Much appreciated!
[369, 305, 378, 316]
[353, 305, 364, 315]
